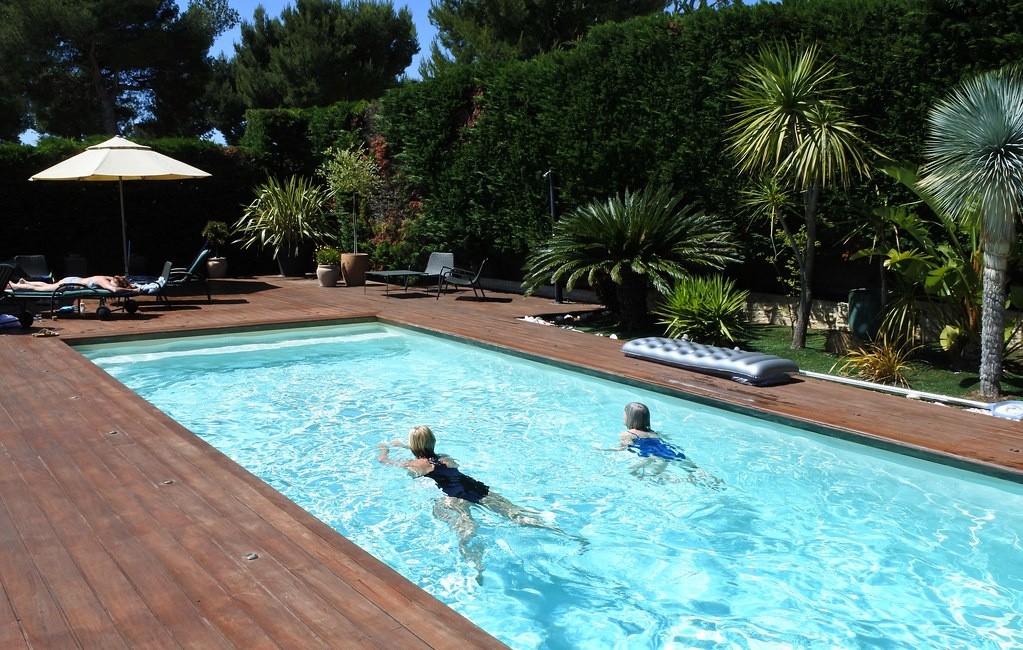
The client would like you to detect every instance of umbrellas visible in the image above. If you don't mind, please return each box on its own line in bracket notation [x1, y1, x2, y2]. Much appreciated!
[29, 135, 212, 275]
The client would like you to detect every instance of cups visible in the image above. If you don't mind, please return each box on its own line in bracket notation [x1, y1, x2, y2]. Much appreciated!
[52, 316, 57, 321]
[33, 313, 42, 323]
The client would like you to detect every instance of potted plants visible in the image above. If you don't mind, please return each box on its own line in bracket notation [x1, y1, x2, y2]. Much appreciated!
[313, 245, 343, 287]
[314, 142, 381, 286]
[201, 221, 230, 278]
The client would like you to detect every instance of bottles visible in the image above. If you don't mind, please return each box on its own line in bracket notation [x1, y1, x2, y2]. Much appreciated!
[80, 300, 85, 312]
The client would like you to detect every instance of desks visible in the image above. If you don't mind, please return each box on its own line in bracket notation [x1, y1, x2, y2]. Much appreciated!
[364, 269, 429, 299]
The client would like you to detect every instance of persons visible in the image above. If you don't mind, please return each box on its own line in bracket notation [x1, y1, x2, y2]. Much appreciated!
[6, 276, 143, 293]
[623, 402, 654, 438]
[409, 426, 442, 463]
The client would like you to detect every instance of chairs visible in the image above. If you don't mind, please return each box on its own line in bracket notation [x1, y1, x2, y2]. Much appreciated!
[404, 251, 458, 292]
[437, 258, 488, 302]
[0, 263, 15, 298]
[117, 262, 173, 311]
[156, 249, 213, 304]
[5, 283, 140, 321]
[14, 255, 49, 276]
